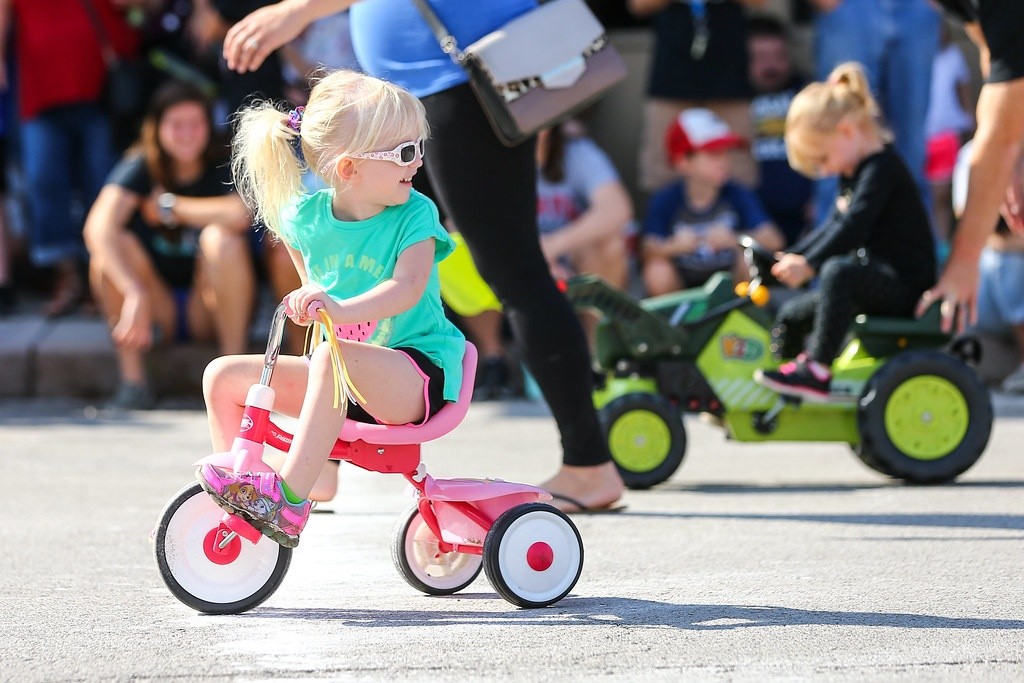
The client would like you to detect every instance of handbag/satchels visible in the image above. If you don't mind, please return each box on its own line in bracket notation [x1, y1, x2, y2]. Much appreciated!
[102, 56, 145, 121]
[461, 0, 627, 148]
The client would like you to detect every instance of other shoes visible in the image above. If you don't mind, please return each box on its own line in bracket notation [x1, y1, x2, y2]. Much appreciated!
[471, 355, 511, 402]
[42, 290, 84, 322]
[97, 382, 158, 414]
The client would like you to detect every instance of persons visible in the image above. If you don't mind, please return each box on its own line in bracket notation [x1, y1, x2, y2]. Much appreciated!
[194, 70, 465, 549]
[750, 64, 941, 405]
[223, 0, 631, 514]
[1, 0, 1024, 407]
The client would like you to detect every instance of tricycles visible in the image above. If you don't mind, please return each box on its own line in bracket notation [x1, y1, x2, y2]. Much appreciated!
[155, 293, 584, 616]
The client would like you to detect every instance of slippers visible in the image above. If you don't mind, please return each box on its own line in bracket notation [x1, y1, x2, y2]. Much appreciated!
[550, 492, 627, 515]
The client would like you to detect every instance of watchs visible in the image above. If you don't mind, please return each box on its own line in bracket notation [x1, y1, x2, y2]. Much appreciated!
[157, 193, 176, 225]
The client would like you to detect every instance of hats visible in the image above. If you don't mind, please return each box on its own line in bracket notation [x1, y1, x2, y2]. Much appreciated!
[665, 107, 751, 167]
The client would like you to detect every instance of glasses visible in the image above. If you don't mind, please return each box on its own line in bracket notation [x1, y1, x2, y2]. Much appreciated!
[320, 136, 425, 174]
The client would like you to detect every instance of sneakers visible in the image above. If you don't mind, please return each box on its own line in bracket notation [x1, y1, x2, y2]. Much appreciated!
[752, 351, 832, 402]
[195, 464, 318, 549]
